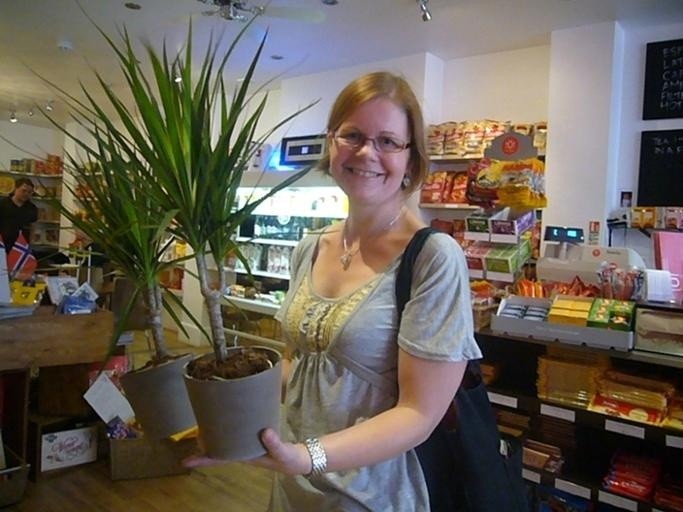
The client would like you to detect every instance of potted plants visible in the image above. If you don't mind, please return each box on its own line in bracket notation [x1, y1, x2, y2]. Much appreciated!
[37, 86, 213, 442]
[25, 1, 324, 462]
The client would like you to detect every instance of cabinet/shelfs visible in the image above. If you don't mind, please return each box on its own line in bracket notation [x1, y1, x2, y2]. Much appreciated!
[470, 329, 683, 511]
[0, 169, 62, 246]
[419, 151, 544, 210]
[206, 213, 348, 349]
[75, 171, 185, 296]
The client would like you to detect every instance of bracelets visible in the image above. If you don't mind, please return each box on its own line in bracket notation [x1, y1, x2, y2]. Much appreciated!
[304, 439, 327, 481]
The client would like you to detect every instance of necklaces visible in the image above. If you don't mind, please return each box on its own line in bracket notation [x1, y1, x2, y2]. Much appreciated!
[340, 204, 404, 266]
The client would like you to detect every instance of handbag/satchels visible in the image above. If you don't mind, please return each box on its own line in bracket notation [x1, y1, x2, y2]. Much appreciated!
[396, 228, 525, 512]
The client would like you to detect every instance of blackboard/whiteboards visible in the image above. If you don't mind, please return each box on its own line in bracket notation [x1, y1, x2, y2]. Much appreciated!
[643, 38, 683, 120]
[637, 129, 683, 207]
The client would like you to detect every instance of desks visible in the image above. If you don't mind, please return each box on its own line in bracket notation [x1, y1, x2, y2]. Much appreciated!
[3, 303, 115, 483]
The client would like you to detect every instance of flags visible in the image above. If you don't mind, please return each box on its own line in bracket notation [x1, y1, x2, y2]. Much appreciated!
[7, 230, 37, 282]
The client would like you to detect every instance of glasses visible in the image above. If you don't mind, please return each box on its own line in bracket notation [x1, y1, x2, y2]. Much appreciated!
[334, 126, 414, 153]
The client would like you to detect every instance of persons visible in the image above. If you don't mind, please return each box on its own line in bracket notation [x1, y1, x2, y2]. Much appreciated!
[0, 177, 39, 253]
[181, 72, 484, 512]
